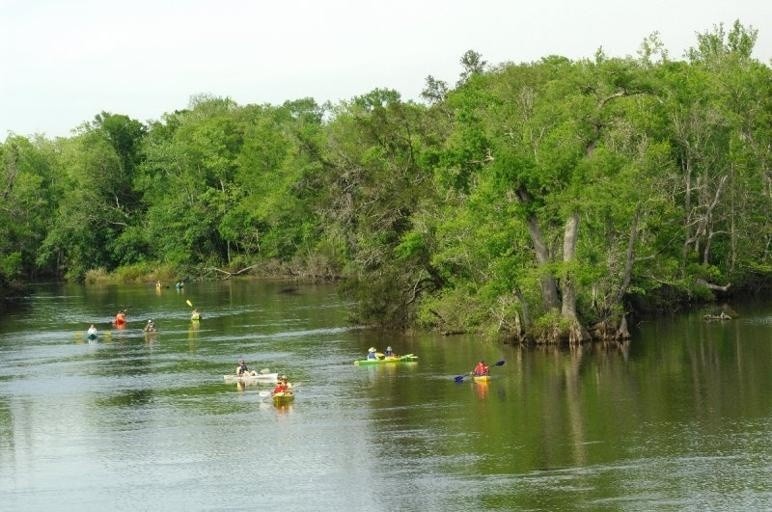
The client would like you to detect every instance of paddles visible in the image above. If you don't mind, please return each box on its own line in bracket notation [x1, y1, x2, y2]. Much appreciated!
[185, 299, 196, 310]
[455, 361, 504, 382]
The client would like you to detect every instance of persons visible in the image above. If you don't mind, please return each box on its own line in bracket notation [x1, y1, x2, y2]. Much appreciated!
[191, 307, 200, 319]
[275, 382, 285, 393]
[367, 347, 377, 359]
[145, 319, 157, 332]
[236, 361, 257, 376]
[115, 310, 126, 328]
[384, 346, 396, 356]
[88, 323, 97, 343]
[280, 375, 294, 393]
[474, 361, 488, 374]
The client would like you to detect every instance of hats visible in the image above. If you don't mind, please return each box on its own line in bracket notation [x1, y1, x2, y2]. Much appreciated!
[387, 346, 392, 351]
[368, 347, 377, 353]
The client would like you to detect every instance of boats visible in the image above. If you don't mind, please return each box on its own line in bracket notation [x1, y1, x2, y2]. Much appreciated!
[271, 392, 294, 408]
[354, 354, 418, 365]
[472, 375, 493, 385]
[85, 280, 200, 342]
[223, 372, 279, 384]
